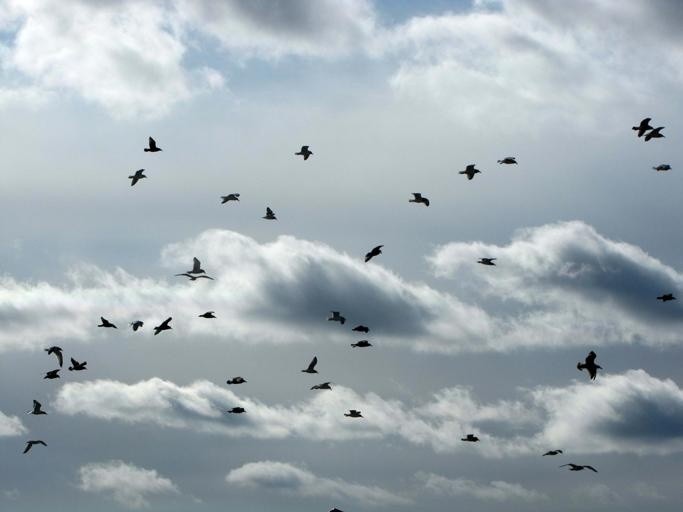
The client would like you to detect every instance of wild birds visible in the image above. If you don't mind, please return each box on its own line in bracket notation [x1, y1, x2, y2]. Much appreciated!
[302, 308, 374, 390]
[364, 244, 382, 264]
[220, 192, 277, 220]
[175, 256, 214, 281]
[44, 345, 87, 380]
[576, 351, 603, 381]
[22, 440, 47, 454]
[476, 257, 497, 267]
[99, 310, 219, 336]
[631, 114, 675, 171]
[295, 143, 313, 162]
[128, 135, 162, 186]
[225, 376, 247, 414]
[344, 409, 364, 418]
[461, 433, 480, 444]
[542, 449, 599, 474]
[25, 400, 48, 416]
[459, 155, 519, 180]
[408, 192, 430, 208]
[656, 293, 677, 303]
